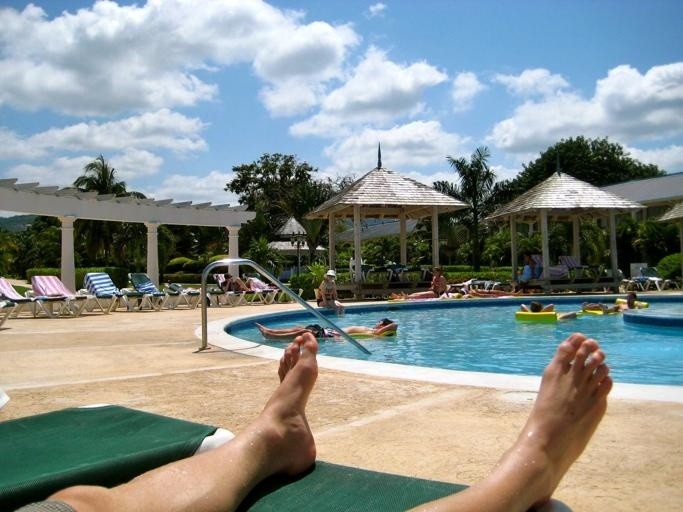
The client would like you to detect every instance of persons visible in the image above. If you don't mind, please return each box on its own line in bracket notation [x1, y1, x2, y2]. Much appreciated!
[385, 267, 448, 300]
[0, 326, 616, 511]
[245, 274, 271, 290]
[219, 274, 256, 294]
[510, 251, 536, 294]
[253, 315, 399, 340]
[348, 249, 364, 285]
[582, 292, 638, 314]
[316, 269, 345, 317]
[519, 302, 577, 321]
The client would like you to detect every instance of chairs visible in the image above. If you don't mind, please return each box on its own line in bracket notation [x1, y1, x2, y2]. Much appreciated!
[348, 258, 426, 282]
[528, 254, 679, 291]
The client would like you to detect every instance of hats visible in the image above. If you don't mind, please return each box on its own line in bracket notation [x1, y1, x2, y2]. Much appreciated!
[324, 270, 337, 281]
